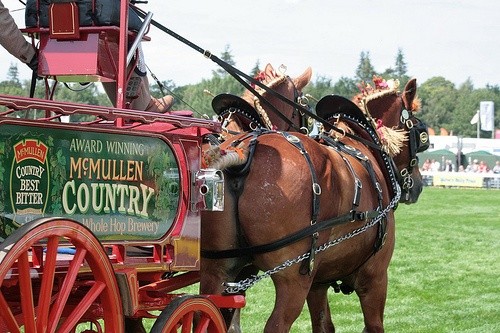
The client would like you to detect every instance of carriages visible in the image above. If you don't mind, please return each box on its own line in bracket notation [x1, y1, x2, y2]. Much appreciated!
[0, 0, 430, 333]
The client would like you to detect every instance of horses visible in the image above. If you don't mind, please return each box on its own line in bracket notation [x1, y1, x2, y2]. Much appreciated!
[192, 63, 425, 333]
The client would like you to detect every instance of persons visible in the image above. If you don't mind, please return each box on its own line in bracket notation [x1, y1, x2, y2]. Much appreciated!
[1, 0, 174, 112]
[421, 155, 500, 176]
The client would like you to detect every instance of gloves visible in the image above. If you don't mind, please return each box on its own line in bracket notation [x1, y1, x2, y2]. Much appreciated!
[28, 48, 44, 80]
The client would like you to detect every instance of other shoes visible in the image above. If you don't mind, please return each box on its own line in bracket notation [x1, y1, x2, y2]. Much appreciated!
[145, 94, 174, 113]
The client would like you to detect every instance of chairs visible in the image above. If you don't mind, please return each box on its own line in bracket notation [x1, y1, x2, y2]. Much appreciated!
[20, 0, 153, 127]
